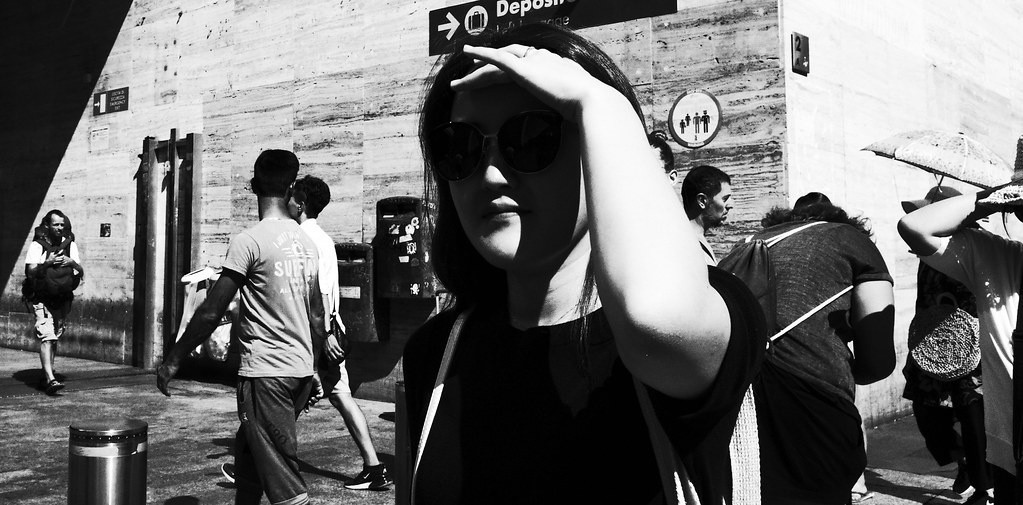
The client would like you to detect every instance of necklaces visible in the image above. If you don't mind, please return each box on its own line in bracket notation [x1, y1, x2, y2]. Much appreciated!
[546, 301, 582, 326]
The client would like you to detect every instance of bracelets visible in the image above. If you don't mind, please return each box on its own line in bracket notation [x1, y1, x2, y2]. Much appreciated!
[324, 331, 334, 339]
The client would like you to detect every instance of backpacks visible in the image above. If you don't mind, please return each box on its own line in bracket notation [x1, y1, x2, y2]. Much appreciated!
[37, 237, 80, 296]
[717, 220, 854, 354]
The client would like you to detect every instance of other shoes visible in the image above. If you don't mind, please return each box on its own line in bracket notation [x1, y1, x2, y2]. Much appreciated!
[221, 462, 236, 483]
[952, 457, 974, 497]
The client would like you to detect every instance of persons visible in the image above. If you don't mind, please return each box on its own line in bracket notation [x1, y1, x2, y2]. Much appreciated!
[23, 209, 84, 396]
[156, 149, 325, 504]
[221, 174, 393, 490]
[648, 129, 1023, 505]
[392, 15, 768, 505]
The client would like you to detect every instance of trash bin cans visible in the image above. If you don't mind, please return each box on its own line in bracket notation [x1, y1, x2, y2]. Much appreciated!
[67, 419, 148, 505]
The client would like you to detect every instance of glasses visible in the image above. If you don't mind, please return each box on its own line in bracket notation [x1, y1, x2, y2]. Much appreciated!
[423, 104, 582, 182]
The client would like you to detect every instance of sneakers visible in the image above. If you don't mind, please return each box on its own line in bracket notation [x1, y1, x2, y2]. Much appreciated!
[344, 462, 393, 490]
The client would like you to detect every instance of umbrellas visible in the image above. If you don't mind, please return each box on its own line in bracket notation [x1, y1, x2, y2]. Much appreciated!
[860, 130, 1015, 210]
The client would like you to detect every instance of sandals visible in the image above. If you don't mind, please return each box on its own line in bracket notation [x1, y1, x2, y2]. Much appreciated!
[46, 379, 65, 395]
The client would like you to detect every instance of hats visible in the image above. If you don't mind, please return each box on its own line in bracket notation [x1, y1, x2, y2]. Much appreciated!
[976, 133, 1023, 208]
[901, 186, 963, 215]
